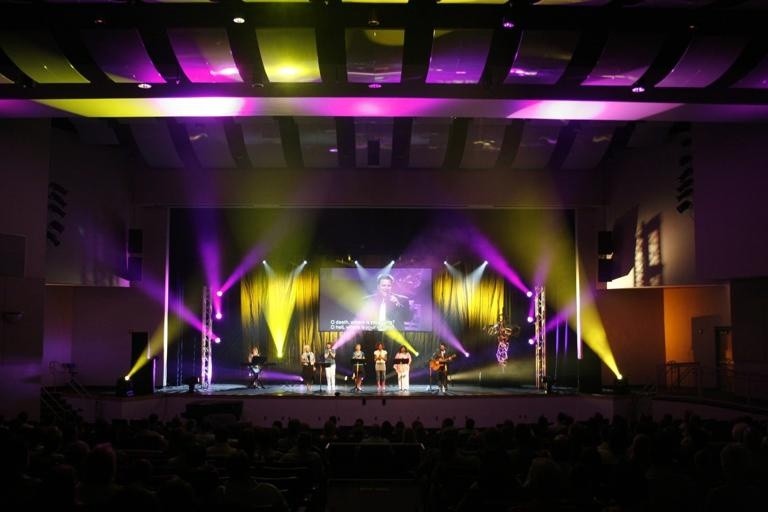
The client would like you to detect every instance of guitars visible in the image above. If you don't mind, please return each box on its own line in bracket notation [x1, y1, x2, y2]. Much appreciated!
[429, 353, 457, 371]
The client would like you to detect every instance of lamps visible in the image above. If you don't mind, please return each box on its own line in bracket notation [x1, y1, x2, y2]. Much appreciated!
[673, 131, 695, 214]
[46, 180, 70, 247]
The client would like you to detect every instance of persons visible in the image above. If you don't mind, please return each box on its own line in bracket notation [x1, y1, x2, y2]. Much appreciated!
[247, 347, 261, 389]
[324, 341, 337, 385]
[300, 344, 316, 385]
[392, 345, 413, 385]
[372, 340, 389, 389]
[349, 343, 368, 387]
[359, 272, 415, 332]
[435, 342, 455, 391]
[1, 411, 766, 512]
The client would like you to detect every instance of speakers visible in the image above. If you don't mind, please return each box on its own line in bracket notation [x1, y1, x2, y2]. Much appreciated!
[131, 359, 153, 394]
[129, 227, 143, 252]
[132, 331, 148, 359]
[578, 357, 601, 393]
[598, 257, 612, 281]
[129, 256, 143, 282]
[597, 231, 613, 253]
